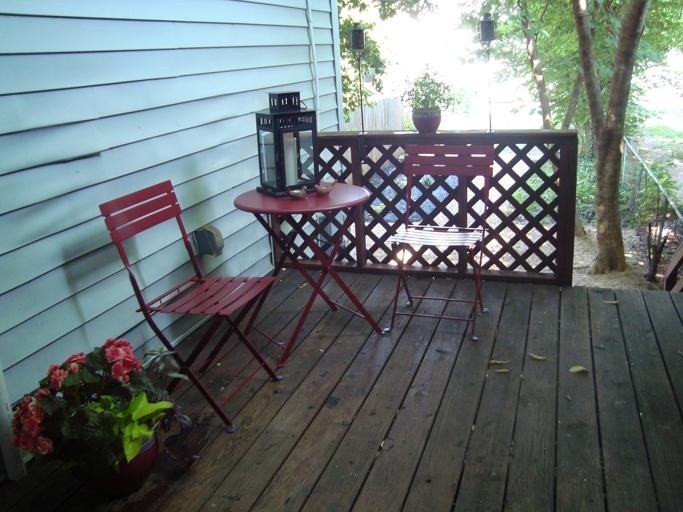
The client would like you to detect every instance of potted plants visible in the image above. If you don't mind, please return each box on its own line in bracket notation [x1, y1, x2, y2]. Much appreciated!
[401, 71, 461, 135]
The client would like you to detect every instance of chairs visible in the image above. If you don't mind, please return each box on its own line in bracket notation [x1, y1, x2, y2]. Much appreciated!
[100, 178, 285, 435]
[382, 145, 497, 340]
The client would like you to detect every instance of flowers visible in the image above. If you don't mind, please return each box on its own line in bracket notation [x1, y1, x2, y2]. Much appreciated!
[8, 336, 202, 478]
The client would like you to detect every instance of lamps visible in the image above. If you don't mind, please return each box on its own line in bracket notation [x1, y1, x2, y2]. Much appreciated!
[477, 12, 497, 137]
[349, 23, 368, 134]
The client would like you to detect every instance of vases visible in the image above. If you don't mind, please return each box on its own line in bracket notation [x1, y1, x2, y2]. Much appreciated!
[76, 434, 159, 500]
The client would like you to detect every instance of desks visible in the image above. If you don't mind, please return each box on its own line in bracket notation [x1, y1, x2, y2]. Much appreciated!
[234, 182, 384, 369]
